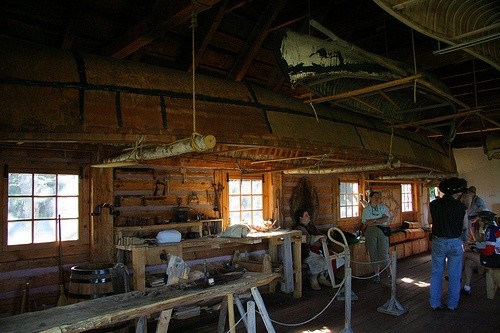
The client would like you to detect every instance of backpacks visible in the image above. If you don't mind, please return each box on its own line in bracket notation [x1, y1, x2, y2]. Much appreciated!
[333, 226, 362, 244]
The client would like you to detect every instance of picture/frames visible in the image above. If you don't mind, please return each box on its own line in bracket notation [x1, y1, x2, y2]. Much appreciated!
[371, 185, 402, 227]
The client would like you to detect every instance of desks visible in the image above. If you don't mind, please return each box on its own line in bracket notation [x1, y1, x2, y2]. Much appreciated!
[114, 229, 303, 298]
[0, 270, 280, 333]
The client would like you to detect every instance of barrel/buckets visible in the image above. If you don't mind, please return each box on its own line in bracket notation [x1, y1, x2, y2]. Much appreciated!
[68, 261, 121, 304]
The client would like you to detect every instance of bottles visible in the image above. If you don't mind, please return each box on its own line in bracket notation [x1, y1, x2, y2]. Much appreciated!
[156, 215, 161, 225]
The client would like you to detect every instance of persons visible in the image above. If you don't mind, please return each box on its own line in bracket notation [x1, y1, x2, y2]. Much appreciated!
[444, 185, 500, 295]
[429, 178, 469, 312]
[361, 192, 391, 282]
[292, 209, 332, 290]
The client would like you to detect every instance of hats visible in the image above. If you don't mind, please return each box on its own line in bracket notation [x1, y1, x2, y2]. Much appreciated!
[468, 186, 476, 193]
[439, 177, 466, 194]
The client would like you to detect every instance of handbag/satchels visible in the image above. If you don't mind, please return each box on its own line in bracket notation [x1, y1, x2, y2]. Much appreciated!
[377, 225, 391, 236]
[219, 222, 250, 239]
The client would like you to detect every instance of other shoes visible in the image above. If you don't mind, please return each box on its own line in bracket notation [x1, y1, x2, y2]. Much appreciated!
[432, 307, 440, 312]
[462, 285, 473, 296]
[374, 273, 380, 283]
[318, 277, 331, 286]
[309, 275, 321, 290]
[447, 307, 457, 312]
